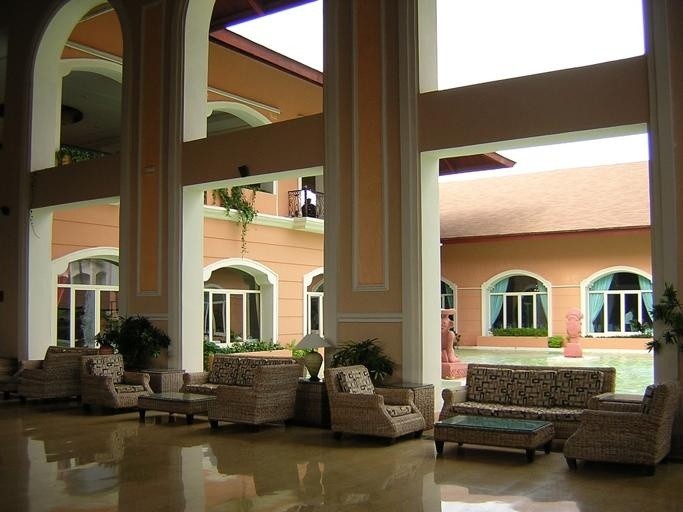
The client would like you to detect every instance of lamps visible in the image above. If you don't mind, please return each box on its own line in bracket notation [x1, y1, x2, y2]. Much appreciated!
[293, 331, 333, 379]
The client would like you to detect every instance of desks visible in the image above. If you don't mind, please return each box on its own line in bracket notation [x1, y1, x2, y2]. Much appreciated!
[292, 379, 330, 429]
[386, 380, 437, 433]
[141, 365, 186, 394]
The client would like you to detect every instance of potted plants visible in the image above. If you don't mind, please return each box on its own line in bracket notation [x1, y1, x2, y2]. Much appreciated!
[93, 330, 118, 355]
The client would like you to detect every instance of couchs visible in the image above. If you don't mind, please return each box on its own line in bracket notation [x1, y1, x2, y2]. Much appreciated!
[324, 364, 428, 443]
[180, 354, 304, 415]
[0, 355, 24, 396]
[79, 353, 155, 409]
[563, 377, 678, 480]
[18, 343, 100, 403]
[435, 357, 622, 449]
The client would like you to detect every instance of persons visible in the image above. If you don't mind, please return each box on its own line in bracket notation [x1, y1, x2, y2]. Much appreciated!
[625, 306, 635, 332]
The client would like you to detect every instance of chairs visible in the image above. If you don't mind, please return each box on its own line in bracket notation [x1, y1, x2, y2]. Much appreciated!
[207, 364, 304, 433]
